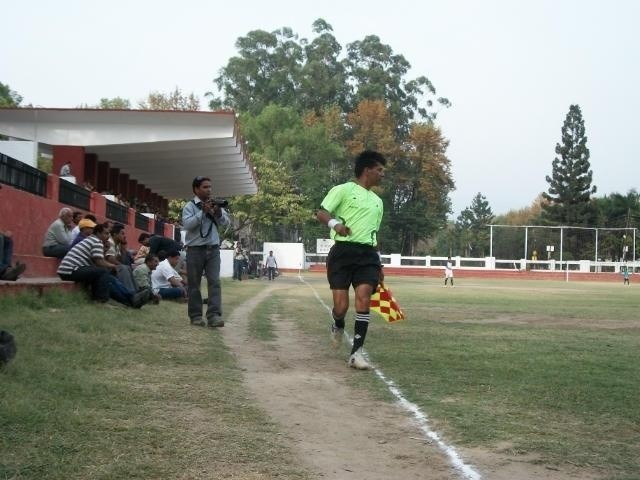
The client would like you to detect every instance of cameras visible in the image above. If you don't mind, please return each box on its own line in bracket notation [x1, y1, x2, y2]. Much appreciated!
[212, 198, 228, 208]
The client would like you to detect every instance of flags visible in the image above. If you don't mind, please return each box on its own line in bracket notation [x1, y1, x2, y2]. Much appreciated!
[370, 279, 404, 324]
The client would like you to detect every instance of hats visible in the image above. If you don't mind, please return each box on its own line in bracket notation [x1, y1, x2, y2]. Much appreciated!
[79, 219, 97, 229]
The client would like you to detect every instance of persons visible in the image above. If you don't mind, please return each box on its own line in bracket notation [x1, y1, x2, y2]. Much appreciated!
[81, 176, 94, 192]
[59, 161, 74, 177]
[115, 192, 179, 228]
[0, 184, 26, 280]
[623, 268, 632, 288]
[42, 207, 188, 308]
[182, 175, 230, 326]
[232, 241, 265, 282]
[221, 234, 233, 249]
[443, 255, 456, 288]
[317, 151, 388, 370]
[265, 251, 278, 280]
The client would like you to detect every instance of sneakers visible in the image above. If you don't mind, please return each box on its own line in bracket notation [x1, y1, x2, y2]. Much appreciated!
[133, 288, 151, 308]
[349, 352, 370, 369]
[331, 325, 345, 348]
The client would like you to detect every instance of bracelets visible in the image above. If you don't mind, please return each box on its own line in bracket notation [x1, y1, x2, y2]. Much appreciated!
[327, 218, 340, 230]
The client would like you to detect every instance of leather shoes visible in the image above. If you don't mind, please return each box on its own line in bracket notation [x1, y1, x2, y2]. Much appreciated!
[2, 263, 26, 282]
[208, 315, 224, 328]
[191, 317, 205, 326]
